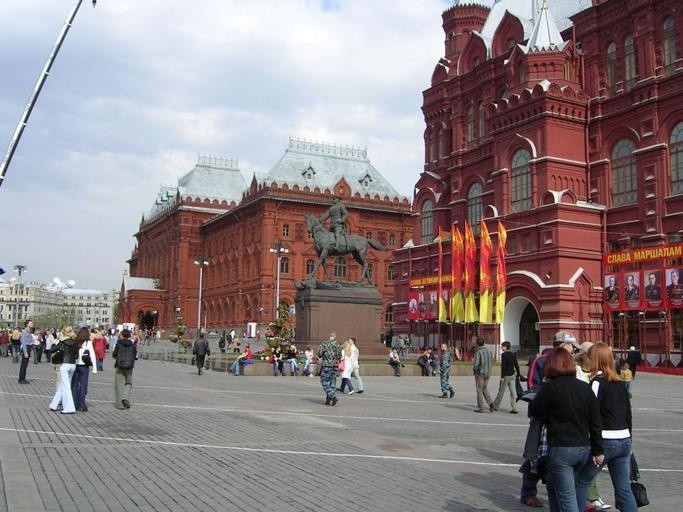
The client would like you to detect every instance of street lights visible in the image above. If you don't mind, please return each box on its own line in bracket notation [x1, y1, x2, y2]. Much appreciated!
[13, 263, 29, 331]
[270, 241, 290, 323]
[193, 255, 211, 339]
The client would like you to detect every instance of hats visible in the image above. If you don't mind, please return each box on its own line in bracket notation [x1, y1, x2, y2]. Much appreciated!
[553, 331, 582, 349]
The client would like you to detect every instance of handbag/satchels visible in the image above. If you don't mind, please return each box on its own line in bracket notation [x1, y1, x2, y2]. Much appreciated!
[615, 482, 649, 512]
[82, 350, 91, 366]
[338, 359, 344, 371]
[52, 348, 63, 364]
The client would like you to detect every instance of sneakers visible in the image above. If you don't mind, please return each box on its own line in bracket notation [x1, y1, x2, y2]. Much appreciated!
[325, 397, 338, 406]
[490, 403, 495, 412]
[474, 408, 489, 413]
[520, 495, 543, 507]
[585, 498, 612, 512]
[439, 390, 455, 398]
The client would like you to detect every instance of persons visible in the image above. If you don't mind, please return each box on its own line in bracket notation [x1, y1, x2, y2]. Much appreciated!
[417, 293, 426, 313]
[191, 333, 210, 376]
[274, 332, 364, 406]
[645, 272, 661, 300]
[429, 292, 438, 315]
[473, 330, 643, 512]
[388, 336, 454, 401]
[605, 276, 619, 302]
[667, 270, 683, 298]
[320, 195, 349, 252]
[216, 328, 261, 377]
[625, 274, 639, 300]
[0, 319, 162, 415]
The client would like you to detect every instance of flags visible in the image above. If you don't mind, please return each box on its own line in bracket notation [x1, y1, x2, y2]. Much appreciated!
[436, 215, 505, 325]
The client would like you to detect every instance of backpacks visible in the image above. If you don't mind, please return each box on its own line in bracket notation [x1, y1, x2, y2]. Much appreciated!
[117, 342, 137, 368]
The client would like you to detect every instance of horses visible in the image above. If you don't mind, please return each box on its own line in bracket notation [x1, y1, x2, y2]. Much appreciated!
[303, 212, 389, 285]
